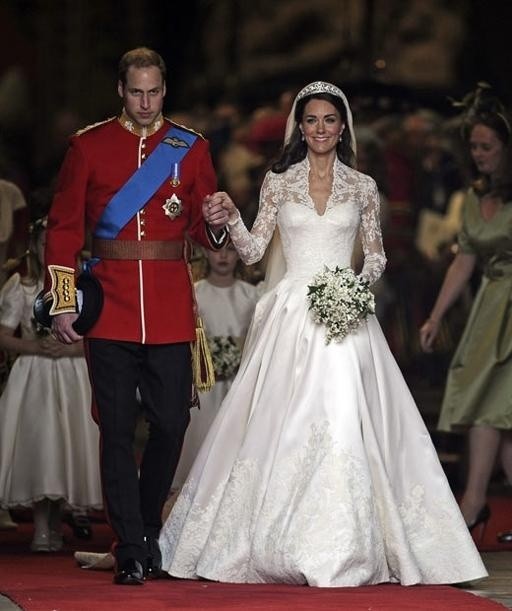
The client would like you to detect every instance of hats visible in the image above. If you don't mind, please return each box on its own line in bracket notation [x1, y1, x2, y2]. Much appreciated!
[32, 273, 102, 340]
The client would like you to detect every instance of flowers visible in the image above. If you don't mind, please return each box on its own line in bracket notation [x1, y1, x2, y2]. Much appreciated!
[305, 262, 380, 349]
[27, 318, 56, 342]
[204, 333, 241, 381]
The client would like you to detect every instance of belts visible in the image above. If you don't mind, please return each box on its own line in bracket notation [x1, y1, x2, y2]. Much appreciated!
[88, 235, 196, 263]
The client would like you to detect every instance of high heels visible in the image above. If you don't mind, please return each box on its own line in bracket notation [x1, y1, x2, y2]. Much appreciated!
[459, 501, 493, 541]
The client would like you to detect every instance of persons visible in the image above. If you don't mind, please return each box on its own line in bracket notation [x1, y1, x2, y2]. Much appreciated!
[41, 44, 231, 587]
[1, 180, 33, 287]
[205, 78, 392, 588]
[158, 229, 260, 489]
[1, 211, 104, 554]
[417, 104, 511, 556]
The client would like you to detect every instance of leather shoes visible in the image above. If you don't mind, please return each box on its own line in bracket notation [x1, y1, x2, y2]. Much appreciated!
[140, 537, 169, 580]
[29, 511, 92, 551]
[113, 545, 145, 586]
